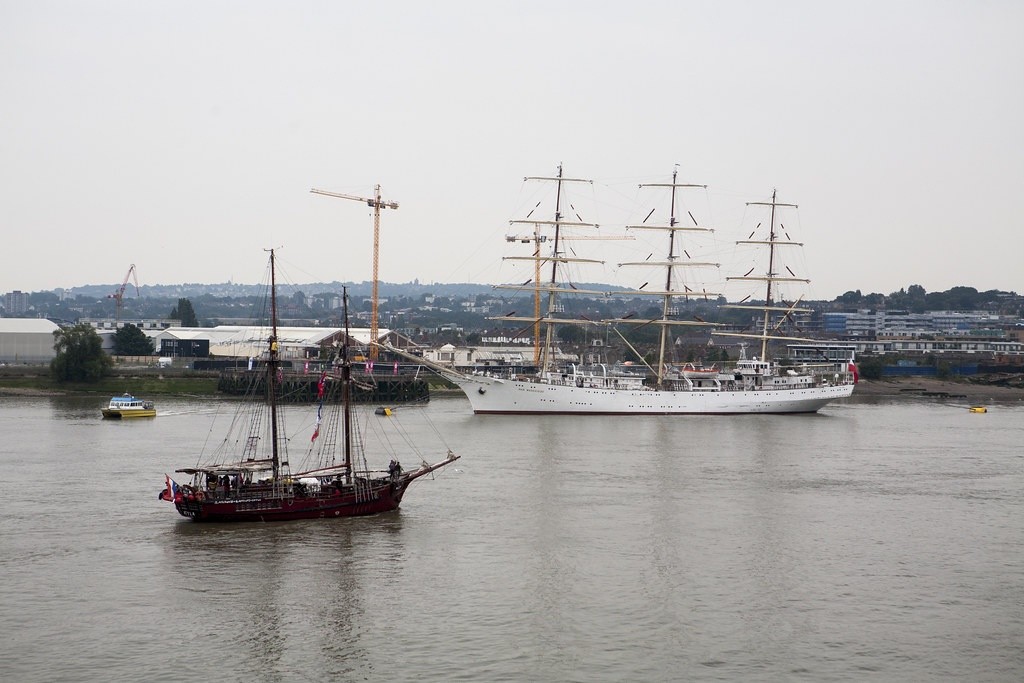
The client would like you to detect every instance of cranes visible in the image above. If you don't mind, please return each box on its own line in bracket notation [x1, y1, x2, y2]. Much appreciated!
[108, 263, 141, 320]
[310, 184, 400, 364]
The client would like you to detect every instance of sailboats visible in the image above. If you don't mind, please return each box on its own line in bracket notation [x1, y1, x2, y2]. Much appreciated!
[371, 163, 860, 416]
[156, 333, 462, 520]
[217, 246, 433, 402]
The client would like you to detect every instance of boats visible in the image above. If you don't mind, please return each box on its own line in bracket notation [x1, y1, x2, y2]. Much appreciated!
[100, 393, 157, 420]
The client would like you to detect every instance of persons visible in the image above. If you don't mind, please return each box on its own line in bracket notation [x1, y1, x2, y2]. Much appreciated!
[207, 474, 250, 498]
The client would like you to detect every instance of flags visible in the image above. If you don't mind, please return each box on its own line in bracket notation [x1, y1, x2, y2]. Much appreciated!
[166, 477, 178, 497]
[311, 373, 326, 441]
[848, 359, 859, 383]
